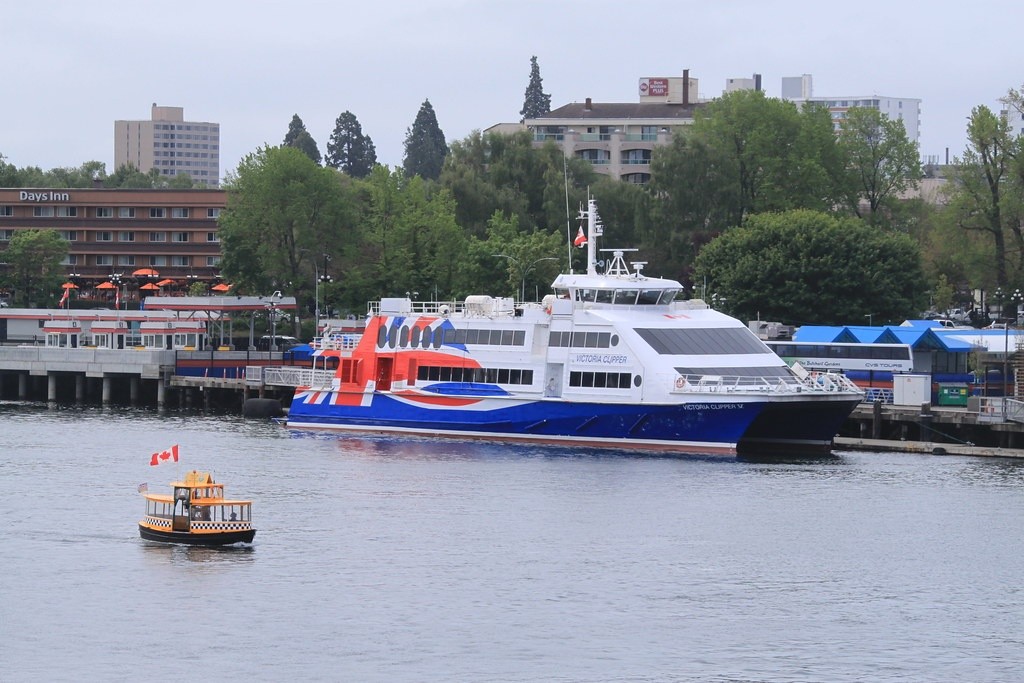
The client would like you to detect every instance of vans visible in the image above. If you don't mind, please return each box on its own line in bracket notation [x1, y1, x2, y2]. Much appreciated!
[259, 336, 304, 351]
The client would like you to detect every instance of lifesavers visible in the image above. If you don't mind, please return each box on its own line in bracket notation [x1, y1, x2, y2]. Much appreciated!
[334, 338, 342, 350]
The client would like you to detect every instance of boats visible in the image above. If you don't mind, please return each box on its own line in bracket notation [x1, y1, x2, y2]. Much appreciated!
[270, 187, 866, 458]
[139, 465, 256, 549]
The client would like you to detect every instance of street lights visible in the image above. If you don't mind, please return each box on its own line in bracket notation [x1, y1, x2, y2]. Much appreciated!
[1011, 289, 1024, 327]
[993, 286, 1005, 321]
[692, 286, 707, 302]
[865, 315, 871, 326]
[109, 274, 121, 302]
[316, 273, 334, 317]
[491, 255, 560, 302]
[270, 289, 283, 351]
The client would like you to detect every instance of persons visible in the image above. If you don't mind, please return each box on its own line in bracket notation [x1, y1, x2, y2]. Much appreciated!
[188, 506, 202, 521]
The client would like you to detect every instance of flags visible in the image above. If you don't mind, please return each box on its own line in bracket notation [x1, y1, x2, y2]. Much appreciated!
[116, 289, 119, 308]
[59, 288, 69, 306]
[574, 226, 587, 246]
[150, 445, 178, 466]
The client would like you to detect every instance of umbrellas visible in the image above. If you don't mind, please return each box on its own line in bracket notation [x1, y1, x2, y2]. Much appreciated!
[140, 283, 160, 289]
[212, 284, 229, 291]
[156, 279, 176, 286]
[96, 282, 118, 288]
[61, 282, 79, 288]
[132, 268, 159, 275]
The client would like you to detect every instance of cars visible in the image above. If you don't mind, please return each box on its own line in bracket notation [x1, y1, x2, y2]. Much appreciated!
[934, 318, 974, 329]
[943, 309, 968, 319]
[963, 309, 1024, 321]
[920, 311, 942, 319]
